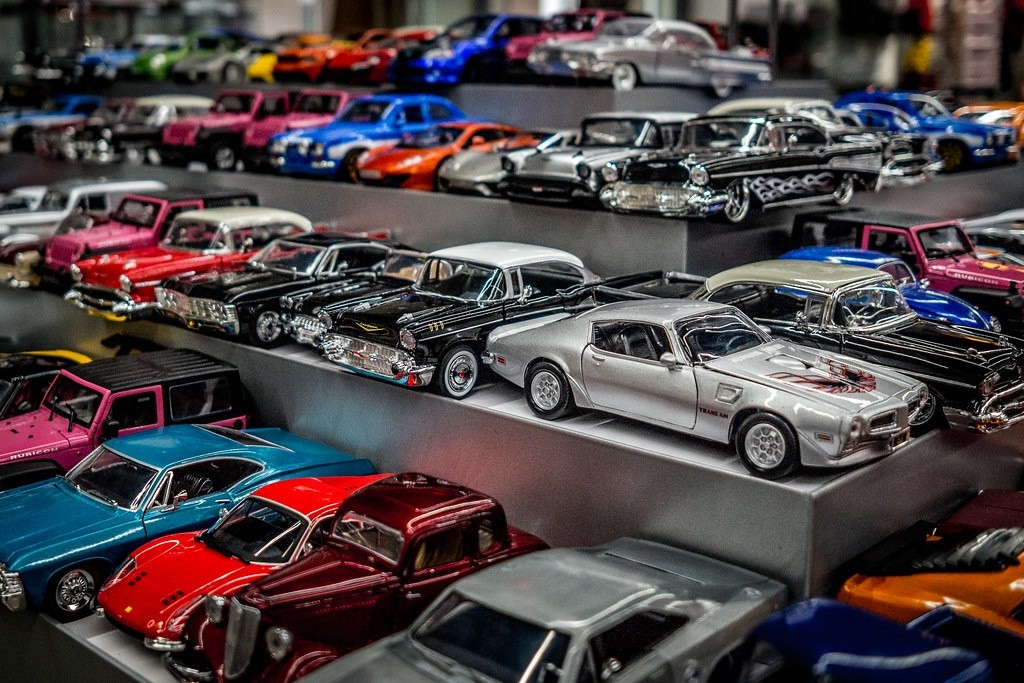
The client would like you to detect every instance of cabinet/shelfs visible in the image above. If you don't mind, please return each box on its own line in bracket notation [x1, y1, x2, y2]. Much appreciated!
[0, 82, 1024, 683]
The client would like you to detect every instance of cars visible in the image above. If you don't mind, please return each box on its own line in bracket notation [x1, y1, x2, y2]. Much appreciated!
[0, 346, 1024, 683]
[0, 92, 1023, 223]
[2, 8, 774, 100]
[0, 177, 1024, 480]
